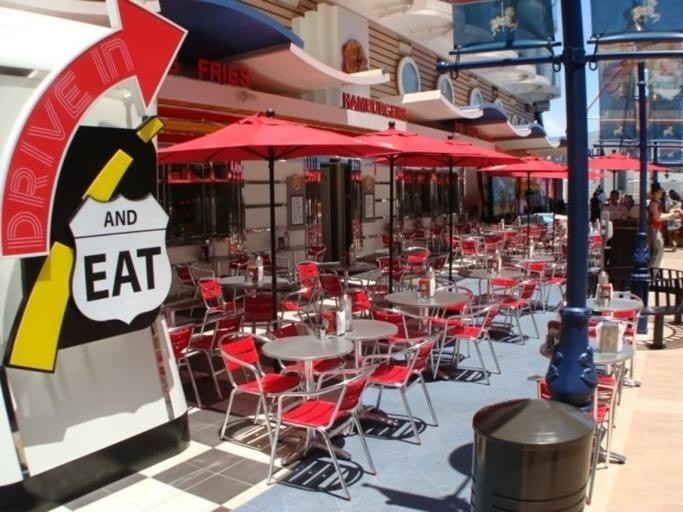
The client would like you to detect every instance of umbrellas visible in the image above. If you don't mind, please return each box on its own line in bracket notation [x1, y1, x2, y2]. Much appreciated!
[475, 155, 607, 239]
[588, 151, 674, 191]
[369, 138, 527, 294]
[159, 108, 405, 375]
[335, 122, 486, 310]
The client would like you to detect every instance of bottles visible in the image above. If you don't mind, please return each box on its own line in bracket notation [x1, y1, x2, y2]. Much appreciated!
[416, 266, 438, 301]
[486, 248, 501, 274]
[245, 255, 264, 282]
[318, 287, 354, 341]
[497, 214, 605, 257]
[277, 235, 284, 248]
[283, 231, 289, 247]
[595, 265, 613, 306]
[339, 244, 356, 265]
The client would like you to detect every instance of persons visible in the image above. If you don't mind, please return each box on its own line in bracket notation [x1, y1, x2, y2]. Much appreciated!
[551, 194, 565, 214]
[510, 191, 529, 221]
[530, 188, 544, 215]
[590, 181, 682, 268]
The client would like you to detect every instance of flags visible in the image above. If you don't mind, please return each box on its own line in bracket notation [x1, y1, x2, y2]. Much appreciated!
[449, 0, 682, 167]
